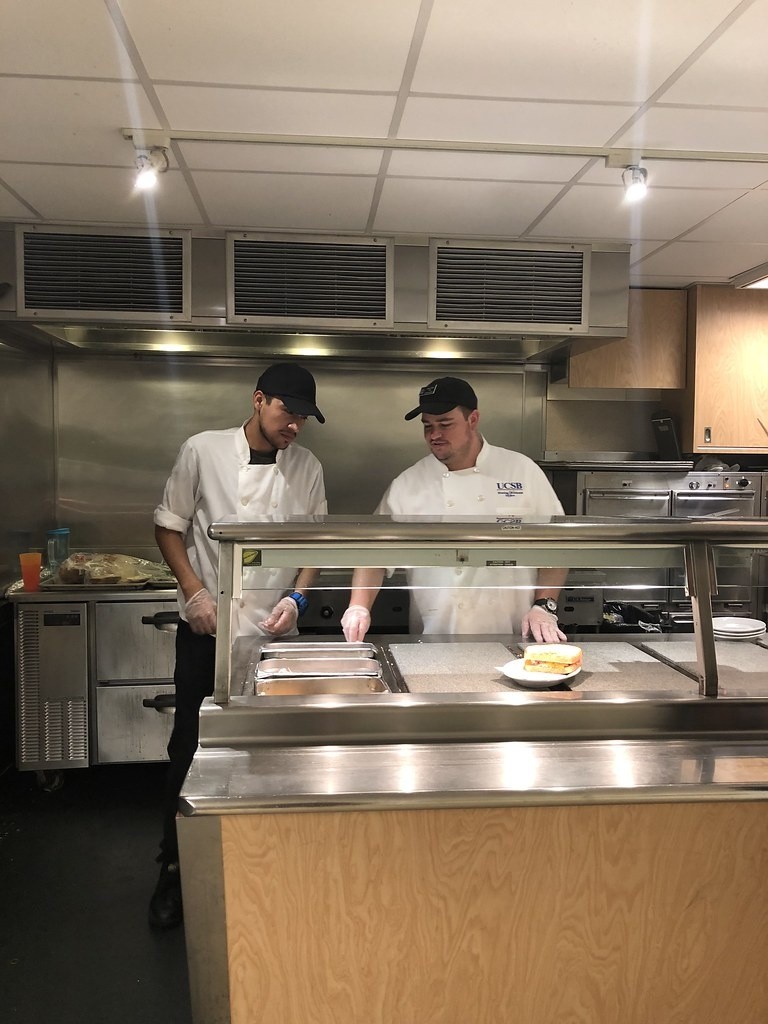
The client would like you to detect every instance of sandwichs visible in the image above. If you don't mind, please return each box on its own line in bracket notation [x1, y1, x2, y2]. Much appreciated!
[523, 643, 582, 674]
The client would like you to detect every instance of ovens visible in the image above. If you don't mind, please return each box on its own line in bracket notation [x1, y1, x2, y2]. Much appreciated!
[551, 469, 767, 635]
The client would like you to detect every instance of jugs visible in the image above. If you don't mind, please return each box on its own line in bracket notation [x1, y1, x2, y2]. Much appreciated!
[46, 527, 72, 577]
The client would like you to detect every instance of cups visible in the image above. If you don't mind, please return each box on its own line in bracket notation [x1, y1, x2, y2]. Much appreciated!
[19, 553, 42, 592]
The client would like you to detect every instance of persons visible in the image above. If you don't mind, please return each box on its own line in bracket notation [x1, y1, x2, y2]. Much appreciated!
[340, 376, 570, 643]
[154, 364, 328, 930]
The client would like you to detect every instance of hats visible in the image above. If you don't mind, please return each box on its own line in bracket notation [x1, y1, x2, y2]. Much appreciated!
[405, 377, 477, 421]
[256, 363, 325, 424]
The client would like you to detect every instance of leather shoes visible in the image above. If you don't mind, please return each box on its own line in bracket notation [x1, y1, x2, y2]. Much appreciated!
[149, 852, 184, 930]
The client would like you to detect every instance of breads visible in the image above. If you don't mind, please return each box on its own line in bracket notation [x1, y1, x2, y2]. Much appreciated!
[126, 575, 152, 583]
[90, 575, 121, 585]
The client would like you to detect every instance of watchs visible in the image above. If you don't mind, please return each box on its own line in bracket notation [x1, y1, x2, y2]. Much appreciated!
[533, 598, 559, 614]
[290, 593, 308, 619]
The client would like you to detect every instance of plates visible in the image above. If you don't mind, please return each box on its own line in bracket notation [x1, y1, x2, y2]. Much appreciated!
[712, 617, 767, 641]
[502, 658, 583, 689]
